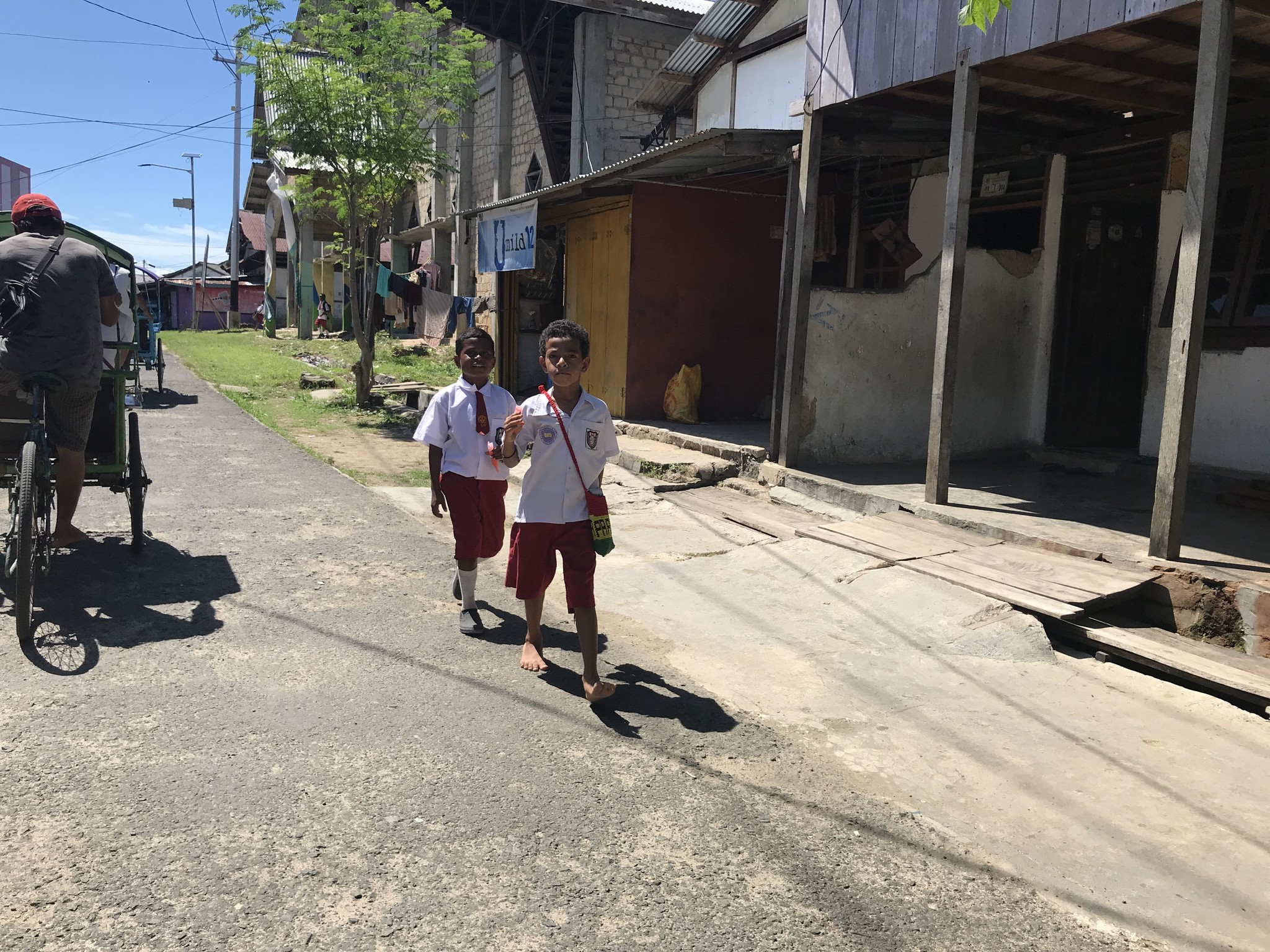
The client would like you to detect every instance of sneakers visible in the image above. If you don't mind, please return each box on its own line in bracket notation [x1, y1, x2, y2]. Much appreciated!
[459, 608, 484, 634]
[452, 569, 463, 600]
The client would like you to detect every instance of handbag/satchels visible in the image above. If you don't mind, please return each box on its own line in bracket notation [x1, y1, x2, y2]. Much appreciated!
[585, 491, 616, 557]
[1, 235, 68, 338]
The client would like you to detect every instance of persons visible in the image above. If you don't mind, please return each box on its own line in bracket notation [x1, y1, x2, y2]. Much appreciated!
[500, 319, 621, 703]
[254, 300, 264, 331]
[100, 257, 154, 371]
[412, 328, 517, 635]
[0, 194, 123, 547]
[314, 293, 331, 338]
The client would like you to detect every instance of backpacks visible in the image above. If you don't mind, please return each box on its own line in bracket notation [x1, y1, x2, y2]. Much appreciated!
[252, 306, 263, 321]
[323, 301, 331, 315]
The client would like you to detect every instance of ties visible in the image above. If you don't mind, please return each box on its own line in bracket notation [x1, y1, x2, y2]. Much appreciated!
[319, 302, 322, 310]
[475, 391, 490, 435]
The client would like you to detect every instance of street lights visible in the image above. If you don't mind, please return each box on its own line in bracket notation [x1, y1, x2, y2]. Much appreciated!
[137, 152, 203, 332]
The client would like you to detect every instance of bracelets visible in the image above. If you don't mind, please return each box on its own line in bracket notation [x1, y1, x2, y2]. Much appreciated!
[500, 445, 517, 458]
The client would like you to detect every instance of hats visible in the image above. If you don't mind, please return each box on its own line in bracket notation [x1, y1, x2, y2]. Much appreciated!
[12, 194, 62, 222]
[319, 293, 326, 297]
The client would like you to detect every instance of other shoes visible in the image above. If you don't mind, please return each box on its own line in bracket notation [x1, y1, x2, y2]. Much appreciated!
[317, 333, 324, 338]
[326, 335, 331, 338]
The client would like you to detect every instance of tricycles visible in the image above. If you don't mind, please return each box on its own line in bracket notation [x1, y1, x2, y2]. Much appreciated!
[0, 208, 154, 636]
[112, 263, 166, 405]
